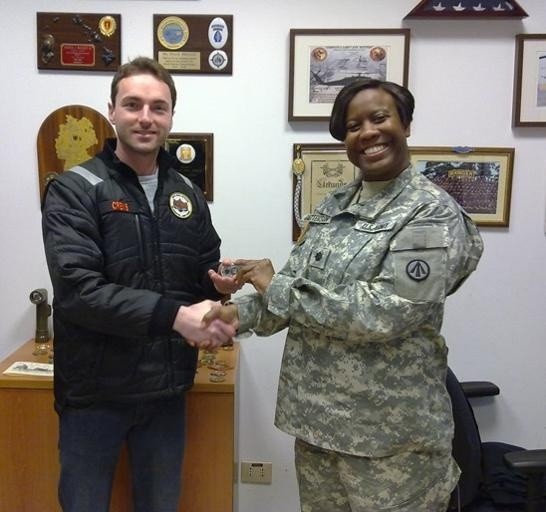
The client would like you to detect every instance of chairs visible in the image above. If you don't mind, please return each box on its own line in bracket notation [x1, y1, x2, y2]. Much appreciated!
[439, 364, 545, 510]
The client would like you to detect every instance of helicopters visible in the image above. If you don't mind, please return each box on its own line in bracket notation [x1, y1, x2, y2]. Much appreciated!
[311, 69, 373, 86]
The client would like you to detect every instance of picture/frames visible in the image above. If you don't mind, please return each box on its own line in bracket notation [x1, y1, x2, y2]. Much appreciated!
[407, 144, 516, 229]
[287, 26, 412, 126]
[507, 31, 546, 130]
[292, 141, 360, 245]
[163, 130, 216, 206]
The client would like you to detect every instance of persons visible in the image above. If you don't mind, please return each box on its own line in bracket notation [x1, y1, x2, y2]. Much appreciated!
[187, 77, 484, 512]
[40, 56, 238, 512]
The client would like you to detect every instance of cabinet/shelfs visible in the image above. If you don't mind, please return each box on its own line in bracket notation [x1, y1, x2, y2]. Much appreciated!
[0, 336, 241, 510]
